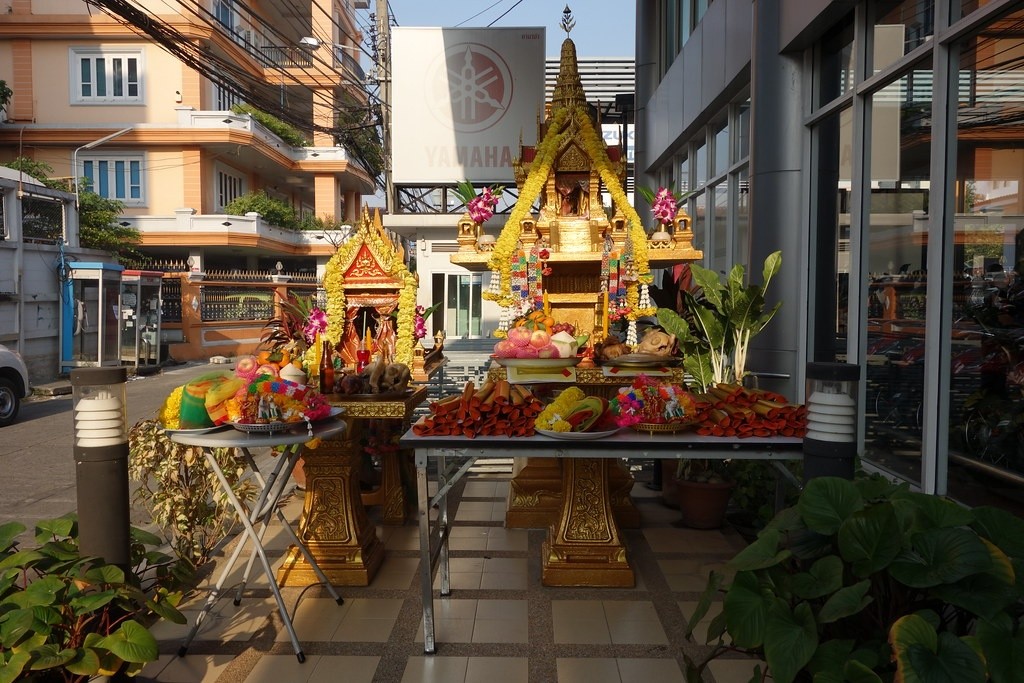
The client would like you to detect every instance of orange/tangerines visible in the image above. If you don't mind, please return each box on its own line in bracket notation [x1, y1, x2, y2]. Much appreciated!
[256, 350, 303, 369]
[515, 311, 555, 334]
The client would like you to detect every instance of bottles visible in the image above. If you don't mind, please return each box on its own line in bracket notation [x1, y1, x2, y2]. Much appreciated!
[320, 341, 334, 395]
[357, 341, 370, 374]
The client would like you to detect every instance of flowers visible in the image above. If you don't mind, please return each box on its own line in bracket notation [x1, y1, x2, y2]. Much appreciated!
[634, 179, 700, 224]
[448, 177, 505, 225]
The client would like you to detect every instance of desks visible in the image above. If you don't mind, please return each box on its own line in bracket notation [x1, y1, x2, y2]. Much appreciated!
[164, 420, 345, 662]
[400, 417, 804, 655]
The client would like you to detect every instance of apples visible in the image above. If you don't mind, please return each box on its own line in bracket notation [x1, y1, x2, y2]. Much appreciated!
[495, 326, 559, 359]
[237, 358, 275, 377]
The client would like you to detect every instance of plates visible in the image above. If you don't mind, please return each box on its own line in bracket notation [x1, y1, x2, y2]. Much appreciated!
[492, 357, 585, 367]
[534, 425, 624, 440]
[599, 356, 674, 368]
[234, 406, 347, 435]
[631, 419, 695, 435]
[165, 424, 227, 436]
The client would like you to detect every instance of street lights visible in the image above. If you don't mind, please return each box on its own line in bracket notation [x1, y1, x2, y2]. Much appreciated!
[299, 36, 393, 243]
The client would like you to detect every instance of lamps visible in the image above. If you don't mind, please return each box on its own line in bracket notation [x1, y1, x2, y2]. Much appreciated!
[187, 257, 195, 271]
[804, 361, 862, 442]
[70, 367, 127, 449]
[276, 262, 284, 275]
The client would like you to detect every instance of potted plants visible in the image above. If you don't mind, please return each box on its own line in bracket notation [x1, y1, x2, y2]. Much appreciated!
[655, 253, 784, 532]
